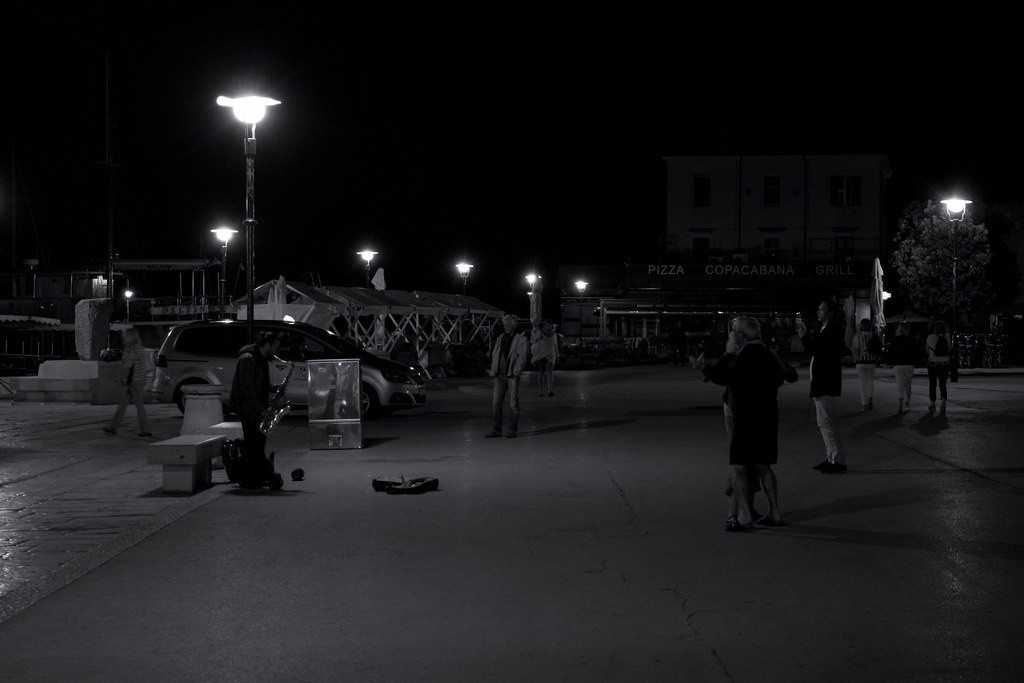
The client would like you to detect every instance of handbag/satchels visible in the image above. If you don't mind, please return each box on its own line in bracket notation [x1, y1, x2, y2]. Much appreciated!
[868, 332, 881, 354]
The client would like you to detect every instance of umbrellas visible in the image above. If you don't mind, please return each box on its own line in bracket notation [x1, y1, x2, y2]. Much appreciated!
[869, 256, 886, 334]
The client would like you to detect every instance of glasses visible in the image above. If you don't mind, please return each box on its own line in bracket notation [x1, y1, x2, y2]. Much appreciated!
[728, 326, 736, 333]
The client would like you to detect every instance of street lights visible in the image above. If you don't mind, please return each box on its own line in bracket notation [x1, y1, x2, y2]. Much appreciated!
[574, 279, 588, 348]
[524, 273, 541, 328]
[940, 194, 973, 381]
[216, 81, 283, 345]
[124, 290, 134, 323]
[455, 262, 473, 296]
[209, 225, 239, 319]
[356, 248, 378, 288]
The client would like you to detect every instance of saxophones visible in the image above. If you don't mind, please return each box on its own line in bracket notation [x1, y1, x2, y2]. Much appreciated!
[257, 354, 296, 436]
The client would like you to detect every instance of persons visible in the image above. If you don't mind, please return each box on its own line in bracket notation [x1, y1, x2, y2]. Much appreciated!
[231, 331, 283, 491]
[103, 327, 152, 436]
[485, 315, 529, 439]
[535, 322, 559, 398]
[689, 301, 960, 532]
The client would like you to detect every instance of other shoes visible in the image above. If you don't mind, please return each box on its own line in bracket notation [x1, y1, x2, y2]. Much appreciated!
[868, 399, 873, 410]
[101, 425, 116, 433]
[725, 507, 783, 532]
[484, 430, 501, 438]
[549, 391, 554, 397]
[896, 408, 903, 416]
[905, 402, 910, 413]
[941, 404, 946, 413]
[138, 432, 152, 437]
[505, 430, 518, 438]
[814, 461, 827, 469]
[929, 405, 936, 411]
[820, 461, 847, 473]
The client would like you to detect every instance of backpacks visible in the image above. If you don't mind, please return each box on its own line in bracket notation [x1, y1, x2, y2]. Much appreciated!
[929, 333, 950, 356]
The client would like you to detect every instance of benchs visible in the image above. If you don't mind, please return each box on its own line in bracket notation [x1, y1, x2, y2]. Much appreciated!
[147, 422, 244, 493]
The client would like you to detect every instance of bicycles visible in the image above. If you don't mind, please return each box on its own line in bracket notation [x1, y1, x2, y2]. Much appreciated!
[948, 332, 1008, 369]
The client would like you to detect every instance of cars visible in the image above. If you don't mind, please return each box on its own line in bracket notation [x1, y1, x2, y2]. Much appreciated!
[150, 319, 427, 421]
